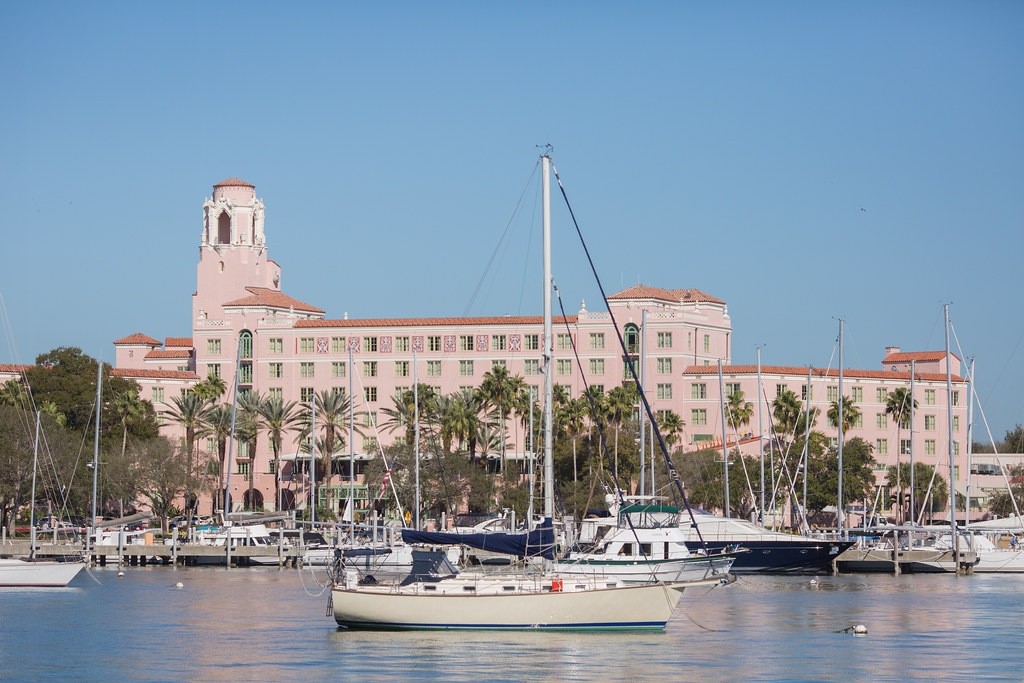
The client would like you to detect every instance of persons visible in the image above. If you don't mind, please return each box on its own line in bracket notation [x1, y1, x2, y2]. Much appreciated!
[404, 509, 411, 528]
[1010, 537, 1019, 552]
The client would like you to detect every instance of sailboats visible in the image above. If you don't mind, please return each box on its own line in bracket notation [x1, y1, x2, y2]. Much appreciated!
[0, 142, 1022, 635]
